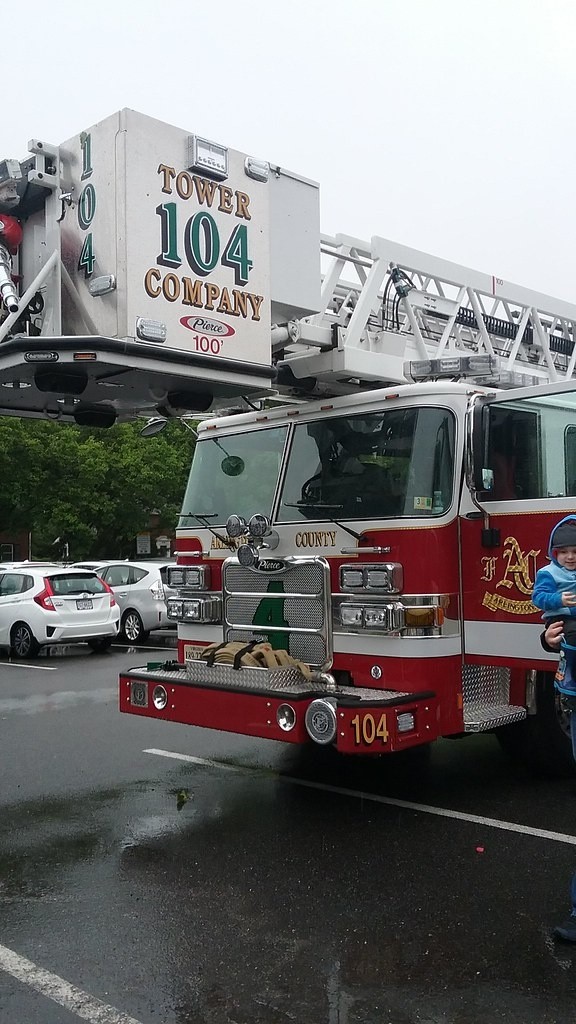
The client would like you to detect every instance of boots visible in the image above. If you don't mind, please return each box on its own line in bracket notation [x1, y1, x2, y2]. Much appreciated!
[553, 642, 576, 696]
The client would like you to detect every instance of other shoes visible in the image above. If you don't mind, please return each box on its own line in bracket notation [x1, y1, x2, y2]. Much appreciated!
[554, 916, 576, 942]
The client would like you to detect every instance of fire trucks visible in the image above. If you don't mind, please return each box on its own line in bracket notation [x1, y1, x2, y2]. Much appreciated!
[0, 107, 576, 778]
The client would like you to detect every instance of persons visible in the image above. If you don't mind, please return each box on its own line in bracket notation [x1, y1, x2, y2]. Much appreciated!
[531, 514, 576, 942]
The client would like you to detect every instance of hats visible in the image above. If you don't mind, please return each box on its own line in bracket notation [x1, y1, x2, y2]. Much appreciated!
[552, 521, 576, 548]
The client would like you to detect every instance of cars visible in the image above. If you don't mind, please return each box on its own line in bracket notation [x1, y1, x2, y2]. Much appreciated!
[0, 559, 140, 588]
[84, 561, 179, 646]
[0, 568, 121, 659]
